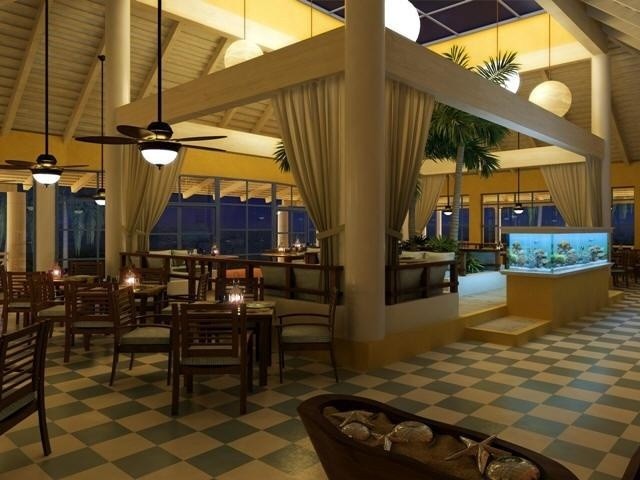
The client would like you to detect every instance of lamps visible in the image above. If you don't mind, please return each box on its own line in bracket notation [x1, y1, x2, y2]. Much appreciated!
[94, 197, 106, 206]
[481, 0, 520, 94]
[32, 170, 63, 188]
[528, 13, 572, 117]
[444, 174, 452, 215]
[138, 142, 183, 170]
[384, 0, 421, 43]
[513, 132, 524, 214]
[224, 0, 264, 68]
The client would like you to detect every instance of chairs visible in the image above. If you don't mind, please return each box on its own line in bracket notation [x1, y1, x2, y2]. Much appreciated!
[0, 316, 54, 457]
[276, 283, 343, 385]
[178, 301, 254, 417]
[107, 284, 173, 388]
[0, 246, 265, 362]
[611, 241, 640, 287]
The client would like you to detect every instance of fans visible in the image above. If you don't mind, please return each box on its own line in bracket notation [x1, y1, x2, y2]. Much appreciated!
[75, 0, 227, 152]
[0, 0, 105, 172]
[73, 55, 105, 200]
